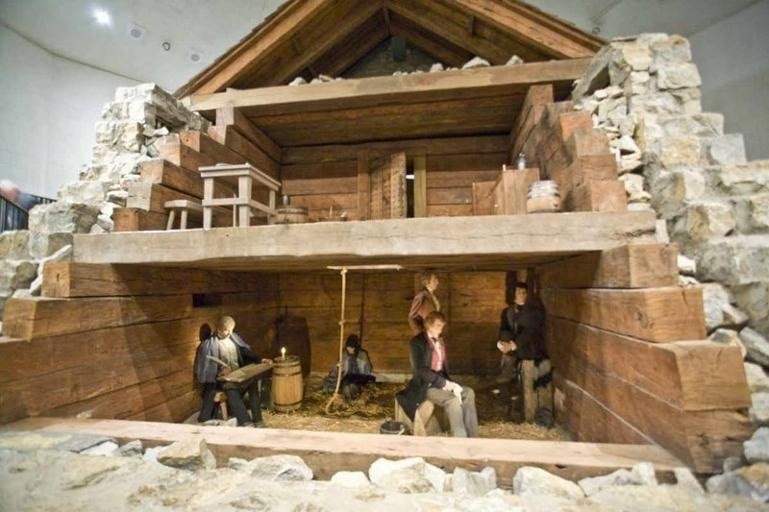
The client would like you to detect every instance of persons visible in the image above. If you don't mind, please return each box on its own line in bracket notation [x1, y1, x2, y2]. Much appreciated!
[396, 310, 478, 436]
[322, 332, 377, 402]
[195, 313, 275, 427]
[409, 268, 446, 335]
[0, 179, 41, 232]
[495, 281, 551, 384]
